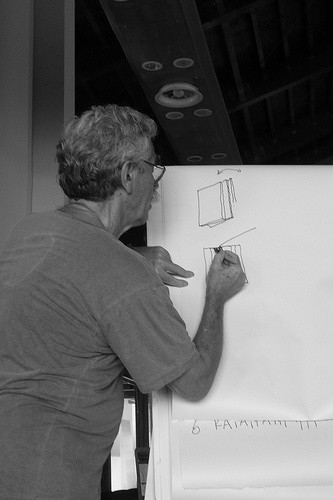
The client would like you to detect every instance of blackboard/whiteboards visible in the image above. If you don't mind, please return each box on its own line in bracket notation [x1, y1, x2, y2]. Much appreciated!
[136, 164, 332, 500]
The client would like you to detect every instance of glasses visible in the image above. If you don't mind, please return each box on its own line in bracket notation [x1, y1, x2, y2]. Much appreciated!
[118, 156, 166, 184]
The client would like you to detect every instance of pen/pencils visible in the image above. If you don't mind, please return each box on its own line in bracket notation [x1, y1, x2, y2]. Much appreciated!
[215, 246, 238, 265]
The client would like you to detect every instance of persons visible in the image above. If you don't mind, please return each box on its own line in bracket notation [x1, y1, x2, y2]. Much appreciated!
[2, 105, 246, 499]
